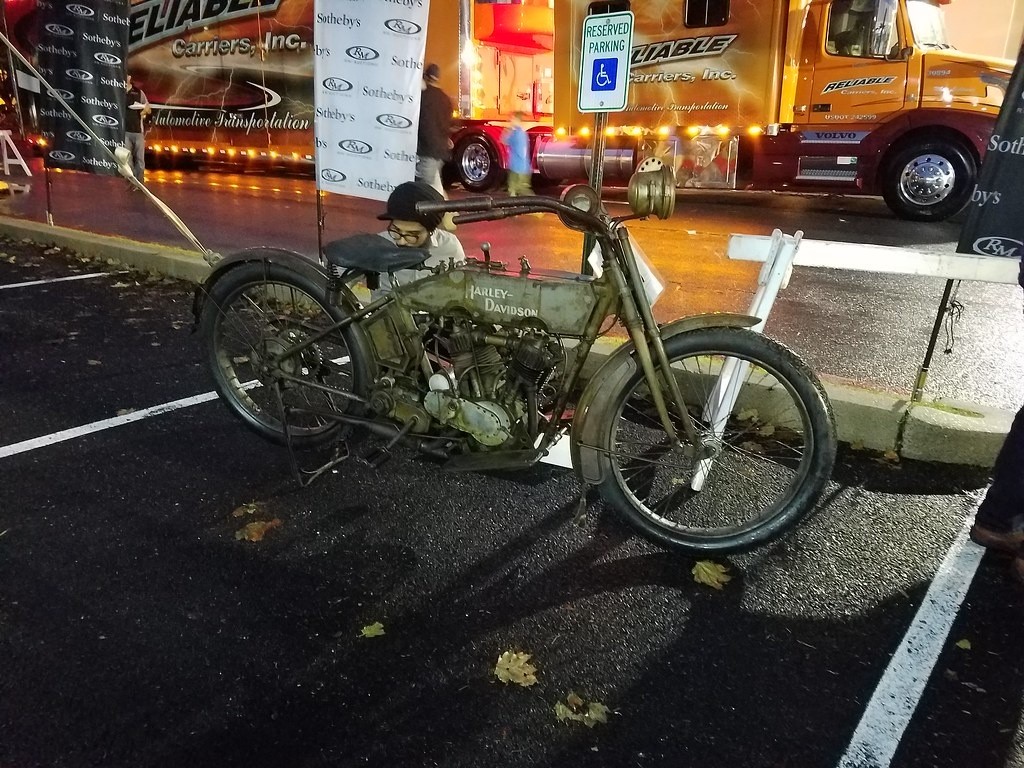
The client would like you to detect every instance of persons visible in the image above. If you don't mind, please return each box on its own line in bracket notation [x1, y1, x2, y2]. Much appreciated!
[971, 246, 1024, 582]
[124, 70, 151, 191]
[500, 111, 543, 217]
[335, 180, 502, 379]
[0, 69, 22, 133]
[415, 63, 460, 230]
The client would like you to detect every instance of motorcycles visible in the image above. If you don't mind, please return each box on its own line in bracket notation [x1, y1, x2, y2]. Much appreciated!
[191, 158, 838, 558]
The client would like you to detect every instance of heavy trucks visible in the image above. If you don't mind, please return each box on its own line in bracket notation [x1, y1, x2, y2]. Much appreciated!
[126, 0, 1017, 220]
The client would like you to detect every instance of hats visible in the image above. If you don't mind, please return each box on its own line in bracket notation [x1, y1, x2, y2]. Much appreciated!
[377, 180, 446, 232]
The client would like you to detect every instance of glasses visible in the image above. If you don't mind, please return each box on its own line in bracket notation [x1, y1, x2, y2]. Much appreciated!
[386, 219, 421, 244]
